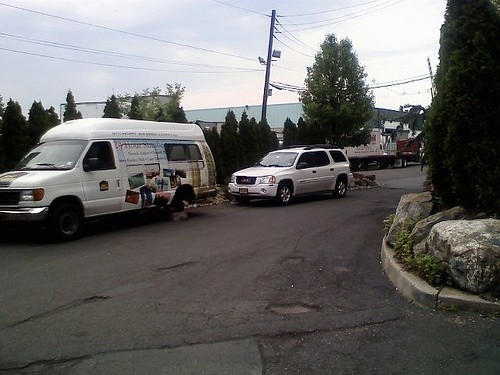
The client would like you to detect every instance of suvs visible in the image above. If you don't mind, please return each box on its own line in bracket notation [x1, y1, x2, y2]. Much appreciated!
[229, 144, 354, 203]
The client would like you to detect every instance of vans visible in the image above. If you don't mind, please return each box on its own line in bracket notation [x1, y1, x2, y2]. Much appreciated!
[1, 115, 220, 236]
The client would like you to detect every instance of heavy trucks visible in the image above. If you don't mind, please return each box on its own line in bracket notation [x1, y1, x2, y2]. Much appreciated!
[339, 134, 424, 171]
